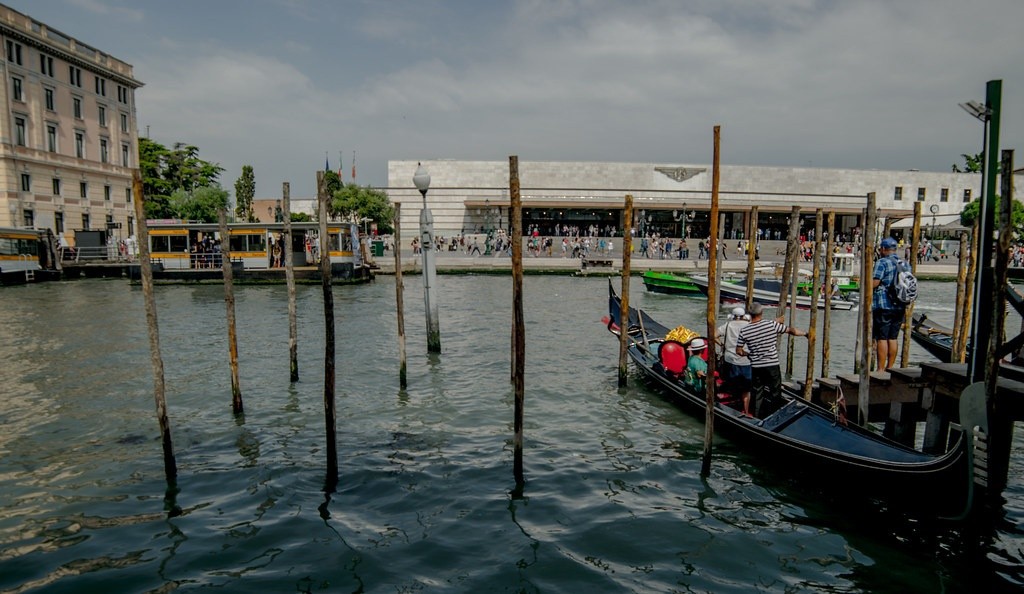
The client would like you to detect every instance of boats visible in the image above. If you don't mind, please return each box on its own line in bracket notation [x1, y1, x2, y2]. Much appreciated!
[0, 226, 64, 285]
[131, 218, 375, 284]
[605, 262, 970, 476]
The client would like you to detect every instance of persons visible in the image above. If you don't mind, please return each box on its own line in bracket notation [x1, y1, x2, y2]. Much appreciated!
[304, 234, 337, 262]
[1006, 242, 1024, 267]
[525, 223, 617, 258]
[262, 234, 285, 268]
[873, 237, 911, 371]
[898, 237, 960, 264]
[684, 302, 809, 418]
[411, 224, 513, 257]
[118, 232, 137, 262]
[367, 232, 395, 250]
[797, 227, 863, 300]
[630, 225, 781, 260]
[189, 233, 223, 268]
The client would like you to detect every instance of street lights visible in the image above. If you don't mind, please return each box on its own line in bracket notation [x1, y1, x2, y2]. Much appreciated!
[413, 159, 444, 354]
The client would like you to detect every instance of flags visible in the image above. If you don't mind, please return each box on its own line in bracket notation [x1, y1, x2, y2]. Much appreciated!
[326, 152, 329, 171]
[338, 154, 342, 178]
[352, 154, 356, 177]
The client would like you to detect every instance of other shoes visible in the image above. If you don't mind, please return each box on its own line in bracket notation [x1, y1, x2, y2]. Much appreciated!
[737, 411, 745, 417]
[746, 414, 754, 419]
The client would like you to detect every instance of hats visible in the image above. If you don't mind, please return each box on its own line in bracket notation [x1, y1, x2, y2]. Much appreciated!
[687, 339, 707, 351]
[732, 308, 745, 316]
[881, 238, 898, 250]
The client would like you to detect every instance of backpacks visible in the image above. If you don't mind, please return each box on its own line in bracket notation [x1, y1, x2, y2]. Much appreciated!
[886, 256, 918, 305]
